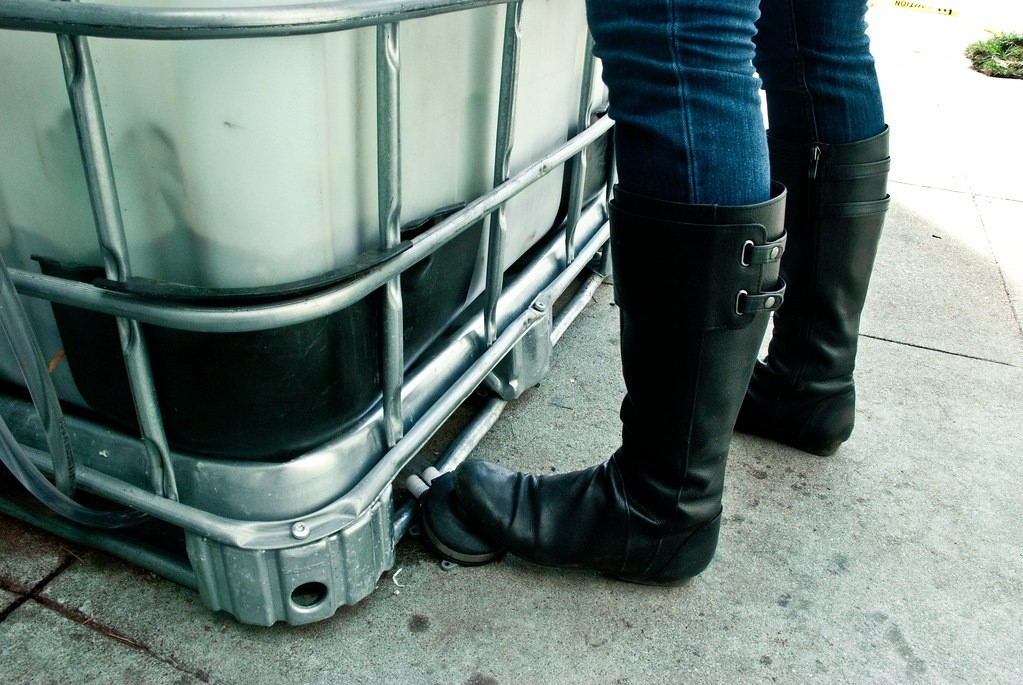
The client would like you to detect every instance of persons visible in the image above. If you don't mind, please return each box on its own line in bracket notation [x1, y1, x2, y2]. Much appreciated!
[447, 1, 891, 588]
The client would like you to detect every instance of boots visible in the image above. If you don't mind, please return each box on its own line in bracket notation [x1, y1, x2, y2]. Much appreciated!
[734, 124, 891, 456]
[453, 184, 787, 587]
